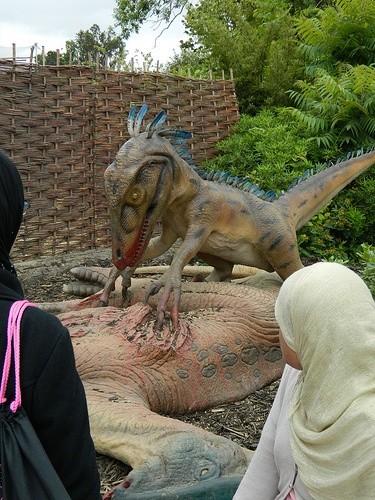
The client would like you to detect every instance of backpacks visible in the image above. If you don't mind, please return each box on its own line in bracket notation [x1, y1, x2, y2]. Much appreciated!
[1, 299, 68, 500]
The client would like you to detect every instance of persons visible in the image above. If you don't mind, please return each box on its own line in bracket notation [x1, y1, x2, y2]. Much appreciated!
[0, 151, 103, 500]
[233, 261, 375, 500]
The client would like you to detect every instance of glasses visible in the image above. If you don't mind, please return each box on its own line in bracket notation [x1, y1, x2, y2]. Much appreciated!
[22, 200, 29, 213]
